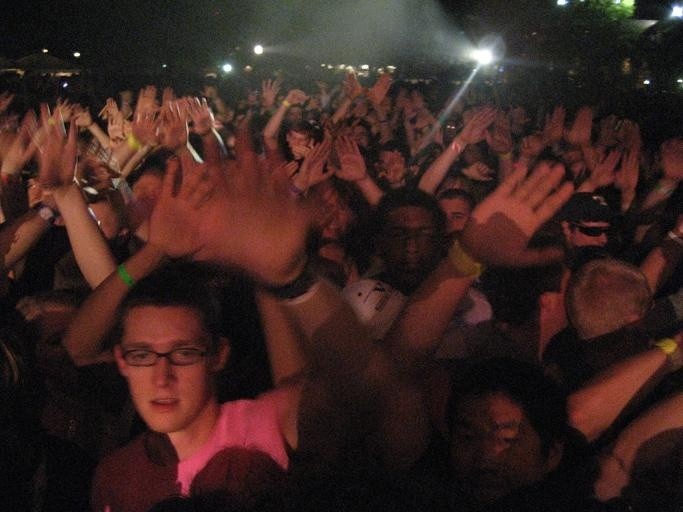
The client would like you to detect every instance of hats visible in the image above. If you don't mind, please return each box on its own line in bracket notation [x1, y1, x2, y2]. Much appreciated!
[556, 192, 625, 229]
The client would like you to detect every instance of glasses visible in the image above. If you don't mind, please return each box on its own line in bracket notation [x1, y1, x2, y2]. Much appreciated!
[570, 219, 616, 238]
[118, 329, 212, 367]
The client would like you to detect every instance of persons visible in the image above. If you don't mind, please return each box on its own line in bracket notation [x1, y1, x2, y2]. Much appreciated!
[0, 73, 683, 512]
